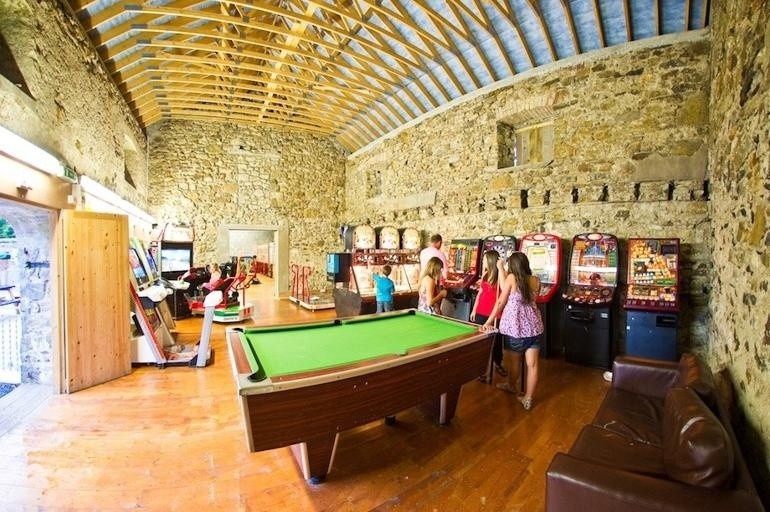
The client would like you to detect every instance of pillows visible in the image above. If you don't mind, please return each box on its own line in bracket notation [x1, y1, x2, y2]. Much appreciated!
[664, 386, 736, 491]
[673, 353, 716, 409]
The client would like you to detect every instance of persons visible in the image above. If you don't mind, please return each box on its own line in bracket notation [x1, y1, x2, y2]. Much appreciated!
[419, 233, 448, 279]
[370, 265, 395, 313]
[470, 250, 545, 411]
[196, 263, 222, 291]
[418, 256, 448, 315]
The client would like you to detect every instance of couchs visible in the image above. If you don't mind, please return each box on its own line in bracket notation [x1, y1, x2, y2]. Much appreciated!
[546, 355, 767, 512]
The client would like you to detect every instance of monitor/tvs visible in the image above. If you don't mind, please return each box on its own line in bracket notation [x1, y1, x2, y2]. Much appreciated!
[160, 246, 192, 274]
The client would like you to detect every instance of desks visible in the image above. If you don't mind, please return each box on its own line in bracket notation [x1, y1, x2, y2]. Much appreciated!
[225, 308, 499, 484]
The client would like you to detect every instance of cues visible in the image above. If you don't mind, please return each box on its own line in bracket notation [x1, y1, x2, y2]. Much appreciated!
[487, 261, 500, 384]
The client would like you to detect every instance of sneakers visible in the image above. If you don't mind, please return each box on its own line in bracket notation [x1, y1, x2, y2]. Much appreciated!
[495, 364, 507, 377]
[496, 383, 516, 393]
[518, 396, 533, 410]
